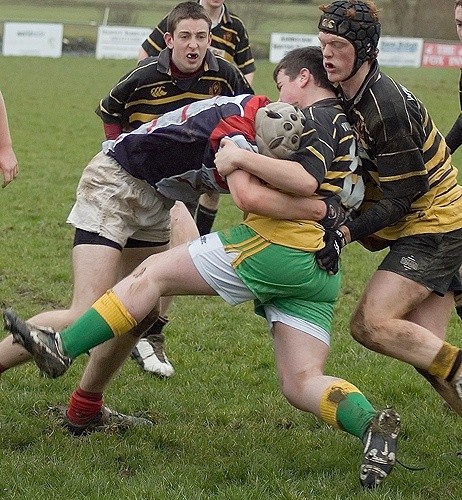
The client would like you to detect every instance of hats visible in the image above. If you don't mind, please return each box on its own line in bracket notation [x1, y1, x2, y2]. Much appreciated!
[318, 0, 382, 69]
[254, 102, 307, 159]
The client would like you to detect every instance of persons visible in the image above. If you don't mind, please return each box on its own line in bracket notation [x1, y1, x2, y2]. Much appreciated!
[314, 0, 462, 418]
[0, 94, 347, 435]
[445, 1, 462, 320]
[0, 91, 19, 188]
[94, 0, 256, 377]
[3, 46, 401, 488]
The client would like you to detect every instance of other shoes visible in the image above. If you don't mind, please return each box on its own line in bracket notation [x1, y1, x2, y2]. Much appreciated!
[457, 451, 462, 460]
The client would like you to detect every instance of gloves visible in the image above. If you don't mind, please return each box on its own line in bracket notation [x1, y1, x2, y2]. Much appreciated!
[315, 229, 347, 276]
[319, 195, 351, 231]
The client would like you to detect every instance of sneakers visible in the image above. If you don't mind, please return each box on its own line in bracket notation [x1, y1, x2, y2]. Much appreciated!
[3, 309, 72, 378]
[86, 349, 92, 356]
[62, 404, 154, 436]
[130, 334, 175, 377]
[359, 409, 401, 490]
[453, 378, 462, 401]
